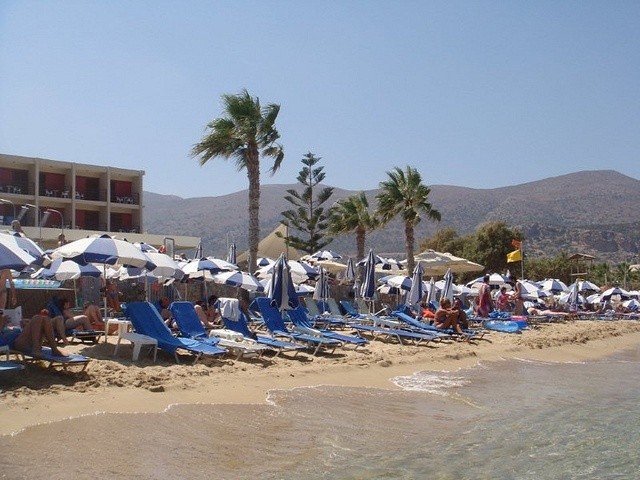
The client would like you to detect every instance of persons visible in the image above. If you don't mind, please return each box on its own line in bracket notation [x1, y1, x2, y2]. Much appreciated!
[64, 301, 93, 331]
[422, 299, 436, 312]
[353, 276, 362, 300]
[451, 298, 468, 328]
[513, 282, 523, 315]
[160, 298, 178, 329]
[0, 310, 68, 357]
[523, 301, 569, 316]
[84, 301, 102, 320]
[12, 220, 25, 235]
[0, 268, 16, 308]
[434, 297, 463, 333]
[39, 292, 93, 343]
[478, 276, 494, 317]
[210, 295, 249, 323]
[377, 281, 407, 294]
[57, 233, 68, 247]
[105, 279, 123, 312]
[465, 296, 478, 318]
[498, 287, 512, 312]
[193, 301, 213, 327]
[414, 299, 435, 317]
[381, 303, 392, 318]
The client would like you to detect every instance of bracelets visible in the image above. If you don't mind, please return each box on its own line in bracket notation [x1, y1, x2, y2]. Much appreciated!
[510, 305, 512, 307]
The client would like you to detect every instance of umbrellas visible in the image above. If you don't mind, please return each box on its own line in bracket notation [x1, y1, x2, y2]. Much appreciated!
[378, 275, 412, 290]
[541, 280, 567, 291]
[215, 271, 264, 291]
[120, 252, 190, 285]
[360, 250, 378, 300]
[312, 250, 341, 259]
[0, 233, 53, 269]
[194, 241, 202, 258]
[270, 252, 300, 312]
[467, 273, 514, 288]
[228, 243, 236, 263]
[383, 258, 403, 269]
[425, 277, 435, 303]
[267, 260, 319, 277]
[134, 241, 156, 252]
[182, 259, 239, 311]
[440, 268, 453, 304]
[517, 280, 551, 298]
[314, 266, 331, 299]
[570, 280, 640, 314]
[30, 248, 101, 307]
[53, 238, 157, 342]
[342, 257, 354, 285]
[254, 258, 274, 266]
[409, 262, 425, 307]
[355, 254, 385, 266]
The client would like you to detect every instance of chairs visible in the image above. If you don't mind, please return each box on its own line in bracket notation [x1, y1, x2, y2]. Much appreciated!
[217, 298, 303, 358]
[77, 322, 105, 330]
[349, 322, 433, 347]
[44, 189, 70, 197]
[304, 298, 347, 331]
[285, 305, 368, 350]
[328, 298, 343, 315]
[169, 302, 270, 361]
[392, 309, 485, 338]
[341, 300, 365, 319]
[528, 309, 638, 322]
[256, 296, 341, 358]
[0, 305, 93, 373]
[116, 197, 134, 204]
[62, 329, 97, 344]
[468, 308, 528, 326]
[125, 301, 226, 363]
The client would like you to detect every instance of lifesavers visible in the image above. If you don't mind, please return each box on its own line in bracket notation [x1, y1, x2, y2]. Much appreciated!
[159, 245, 165, 252]
[485, 320, 519, 332]
[510, 316, 527, 329]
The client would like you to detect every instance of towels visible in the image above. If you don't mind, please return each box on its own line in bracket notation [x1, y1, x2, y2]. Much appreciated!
[218, 296, 241, 323]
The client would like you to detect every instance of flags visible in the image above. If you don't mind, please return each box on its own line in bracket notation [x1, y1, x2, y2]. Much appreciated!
[506, 249, 522, 262]
[512, 240, 522, 249]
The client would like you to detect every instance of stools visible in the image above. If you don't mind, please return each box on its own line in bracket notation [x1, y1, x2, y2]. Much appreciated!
[105, 318, 132, 342]
[114, 332, 159, 364]
